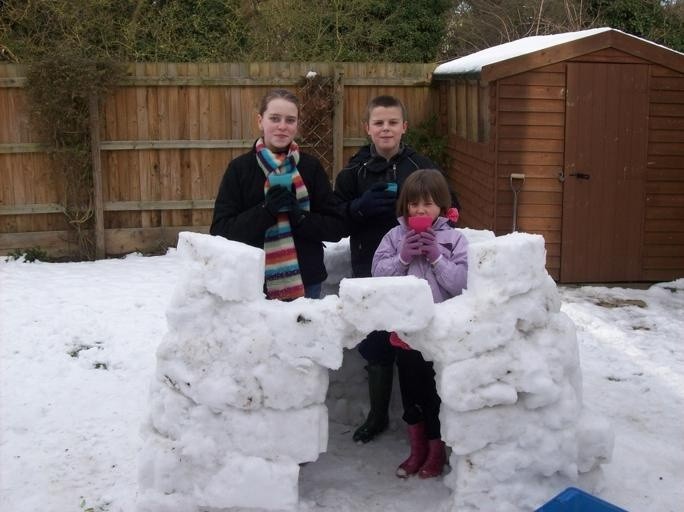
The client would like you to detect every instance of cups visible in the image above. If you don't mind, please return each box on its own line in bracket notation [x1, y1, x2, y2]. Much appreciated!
[406, 216, 434, 256]
[385, 182, 398, 193]
[267, 171, 296, 214]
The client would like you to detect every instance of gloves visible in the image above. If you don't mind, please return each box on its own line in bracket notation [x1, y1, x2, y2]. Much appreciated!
[352, 183, 397, 221]
[265, 182, 307, 228]
[400, 229, 425, 265]
[421, 228, 442, 265]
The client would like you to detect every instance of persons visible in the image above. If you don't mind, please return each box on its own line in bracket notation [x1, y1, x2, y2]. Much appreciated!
[334, 98, 460, 445]
[371, 169, 471, 478]
[209, 90, 341, 304]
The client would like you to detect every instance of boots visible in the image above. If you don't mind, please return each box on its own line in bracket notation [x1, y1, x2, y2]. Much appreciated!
[353, 360, 445, 478]
